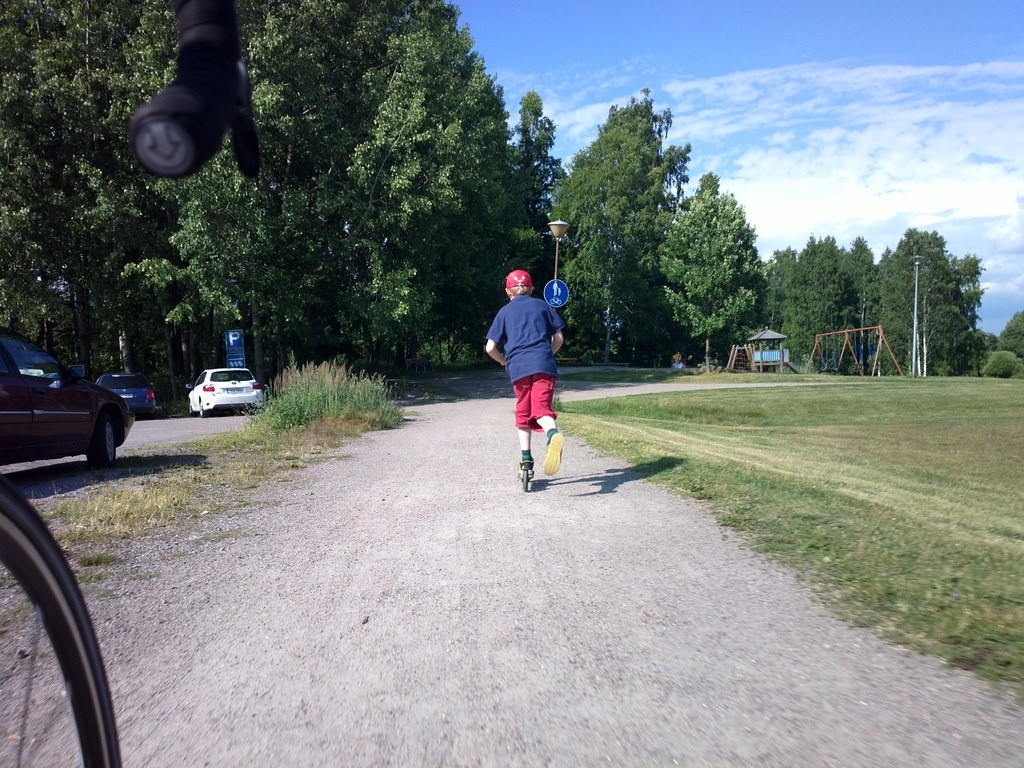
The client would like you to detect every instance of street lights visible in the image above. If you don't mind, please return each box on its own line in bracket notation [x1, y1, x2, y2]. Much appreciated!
[911, 254, 924, 378]
[547, 218, 571, 344]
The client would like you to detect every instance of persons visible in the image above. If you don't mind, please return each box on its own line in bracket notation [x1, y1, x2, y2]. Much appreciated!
[485, 270, 565, 480]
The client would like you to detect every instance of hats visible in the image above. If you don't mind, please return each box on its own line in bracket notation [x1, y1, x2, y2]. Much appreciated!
[505, 269, 533, 288]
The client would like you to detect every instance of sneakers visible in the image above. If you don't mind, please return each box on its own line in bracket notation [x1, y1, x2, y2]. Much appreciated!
[519, 457, 534, 471]
[542, 432, 564, 476]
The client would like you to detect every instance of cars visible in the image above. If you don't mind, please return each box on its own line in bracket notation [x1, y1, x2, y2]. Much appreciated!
[186, 368, 264, 418]
[95, 373, 156, 420]
[0, 325, 136, 468]
